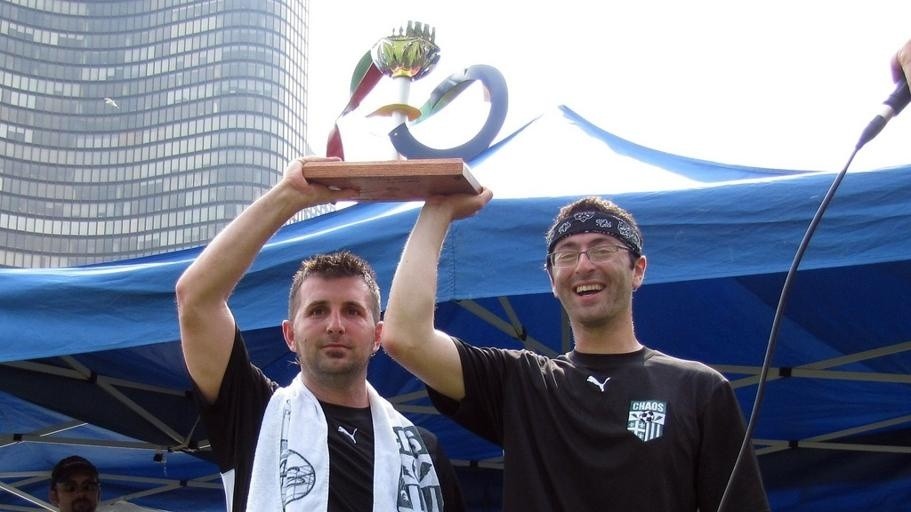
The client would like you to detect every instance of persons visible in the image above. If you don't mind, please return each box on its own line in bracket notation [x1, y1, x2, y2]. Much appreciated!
[380, 186, 770, 512]
[174, 155, 465, 510]
[46, 456, 103, 511]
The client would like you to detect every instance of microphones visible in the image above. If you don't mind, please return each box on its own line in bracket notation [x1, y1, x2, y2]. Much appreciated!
[863, 79, 911, 145]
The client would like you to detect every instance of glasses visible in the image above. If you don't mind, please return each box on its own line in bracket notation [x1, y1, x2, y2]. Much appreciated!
[550, 245, 640, 269]
[57, 480, 99, 493]
